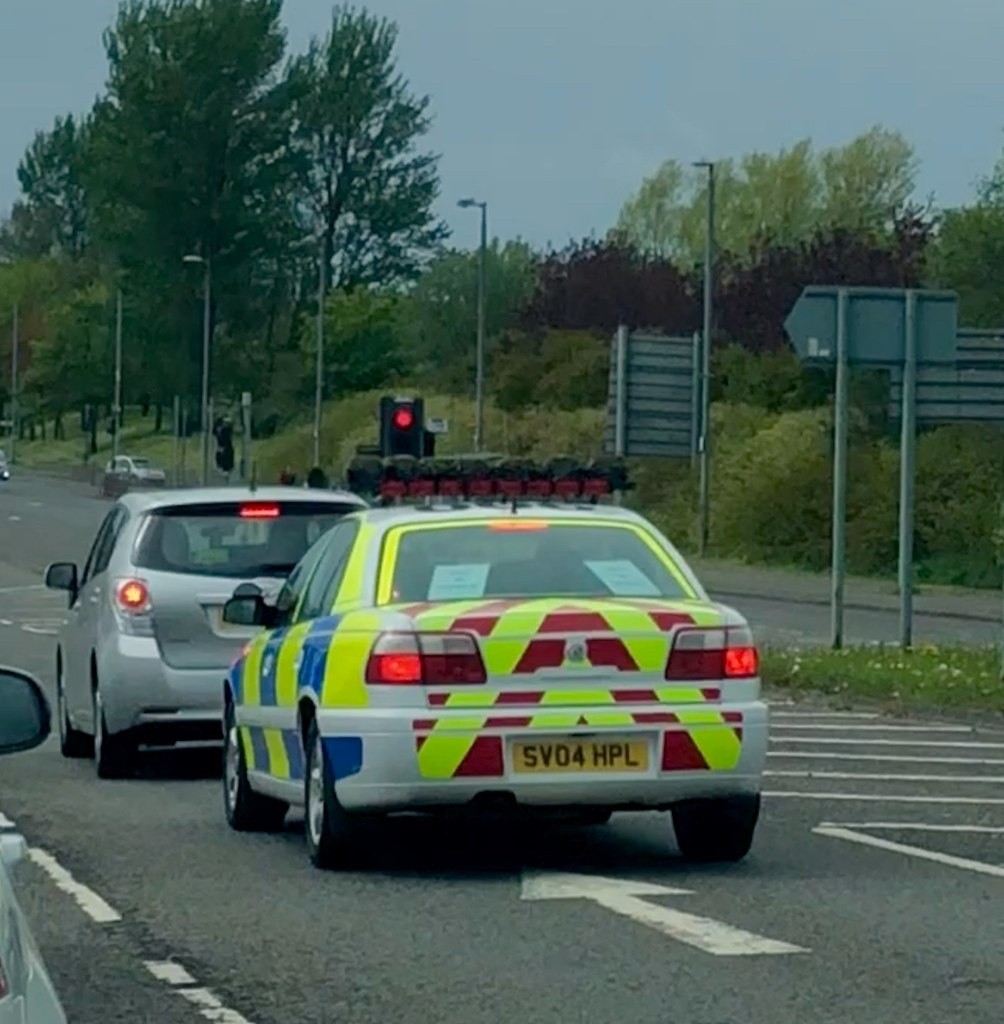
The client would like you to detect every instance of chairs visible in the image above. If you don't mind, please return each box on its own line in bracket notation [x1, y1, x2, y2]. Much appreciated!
[150, 521, 187, 563]
[269, 517, 315, 562]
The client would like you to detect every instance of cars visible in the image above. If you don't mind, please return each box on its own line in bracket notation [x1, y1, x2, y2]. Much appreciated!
[0, 449, 11, 479]
[222, 454, 770, 870]
[0, 665, 67, 1024]
[105, 455, 166, 487]
[43, 482, 372, 777]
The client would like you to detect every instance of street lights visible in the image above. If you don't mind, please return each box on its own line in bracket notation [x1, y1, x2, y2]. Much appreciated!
[288, 238, 326, 468]
[114, 270, 133, 455]
[457, 197, 487, 454]
[183, 255, 212, 486]
[692, 162, 715, 558]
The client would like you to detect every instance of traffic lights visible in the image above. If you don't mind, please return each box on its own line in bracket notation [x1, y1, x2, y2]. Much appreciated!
[379, 394, 424, 457]
